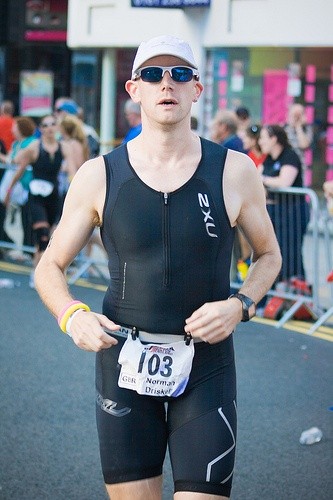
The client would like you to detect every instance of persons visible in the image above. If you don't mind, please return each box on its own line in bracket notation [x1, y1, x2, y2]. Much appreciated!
[6, 114, 77, 289]
[191, 106, 269, 308]
[121, 99, 142, 144]
[33, 36, 282, 500]
[0, 97, 109, 278]
[257, 124, 309, 291]
[284, 103, 311, 184]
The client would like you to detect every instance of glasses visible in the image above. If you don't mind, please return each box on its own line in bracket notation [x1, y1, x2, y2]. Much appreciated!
[131, 66, 199, 82]
[39, 122, 56, 128]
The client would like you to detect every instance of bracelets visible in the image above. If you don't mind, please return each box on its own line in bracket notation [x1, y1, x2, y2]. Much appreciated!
[6, 187, 12, 194]
[57, 300, 91, 338]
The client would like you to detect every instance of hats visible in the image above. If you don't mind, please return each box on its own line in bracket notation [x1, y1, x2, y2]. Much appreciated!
[56, 101, 78, 116]
[131, 36, 197, 77]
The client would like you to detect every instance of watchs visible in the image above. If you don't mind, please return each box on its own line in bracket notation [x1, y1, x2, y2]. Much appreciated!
[228, 293, 256, 322]
